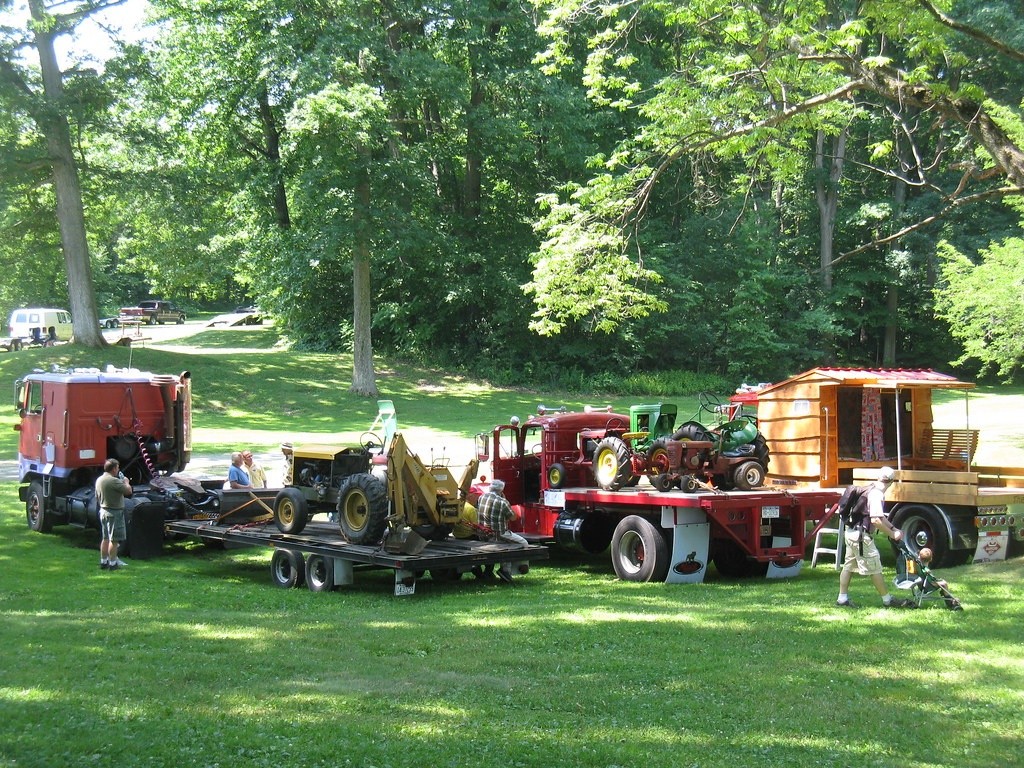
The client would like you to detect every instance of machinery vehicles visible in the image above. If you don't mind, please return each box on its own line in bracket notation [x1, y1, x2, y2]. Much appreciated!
[213, 433, 480, 555]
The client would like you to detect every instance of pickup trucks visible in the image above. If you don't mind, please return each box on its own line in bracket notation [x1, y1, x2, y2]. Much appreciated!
[121, 300, 186, 329]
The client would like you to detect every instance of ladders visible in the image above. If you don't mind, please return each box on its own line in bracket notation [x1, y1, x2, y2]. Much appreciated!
[810, 503, 844, 570]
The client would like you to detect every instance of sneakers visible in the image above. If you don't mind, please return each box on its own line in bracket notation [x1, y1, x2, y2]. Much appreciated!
[836, 599, 859, 609]
[884, 596, 906, 607]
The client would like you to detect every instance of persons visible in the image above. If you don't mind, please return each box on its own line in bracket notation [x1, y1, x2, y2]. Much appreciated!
[228, 452, 253, 488]
[242, 451, 267, 488]
[280, 441, 293, 487]
[919, 547, 955, 607]
[835, 465, 908, 608]
[95, 458, 132, 570]
[478, 480, 527, 583]
[452, 487, 496, 577]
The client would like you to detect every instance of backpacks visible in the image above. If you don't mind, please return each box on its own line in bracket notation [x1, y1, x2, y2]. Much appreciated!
[837, 480, 876, 529]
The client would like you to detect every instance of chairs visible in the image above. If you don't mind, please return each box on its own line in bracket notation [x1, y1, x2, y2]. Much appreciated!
[711, 420, 758, 450]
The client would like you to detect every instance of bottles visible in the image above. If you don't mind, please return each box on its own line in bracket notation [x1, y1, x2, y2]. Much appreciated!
[119, 471, 125, 480]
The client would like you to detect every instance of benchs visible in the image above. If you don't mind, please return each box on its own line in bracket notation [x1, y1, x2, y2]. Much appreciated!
[904, 428, 981, 473]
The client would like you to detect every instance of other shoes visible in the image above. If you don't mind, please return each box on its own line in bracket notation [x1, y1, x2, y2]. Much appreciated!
[519, 564, 529, 574]
[484, 568, 496, 580]
[471, 566, 484, 578]
[497, 568, 512, 582]
[947, 599, 960, 607]
[109, 562, 121, 571]
[100, 560, 109, 569]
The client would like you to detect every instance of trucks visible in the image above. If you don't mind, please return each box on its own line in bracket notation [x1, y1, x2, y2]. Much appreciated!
[462, 403, 841, 586]
[14, 364, 551, 592]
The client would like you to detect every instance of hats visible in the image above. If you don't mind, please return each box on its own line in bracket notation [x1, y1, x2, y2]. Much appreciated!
[880, 465, 895, 480]
[466, 486, 484, 494]
[281, 442, 293, 452]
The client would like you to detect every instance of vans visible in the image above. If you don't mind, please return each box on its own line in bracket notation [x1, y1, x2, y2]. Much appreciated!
[9, 308, 74, 342]
